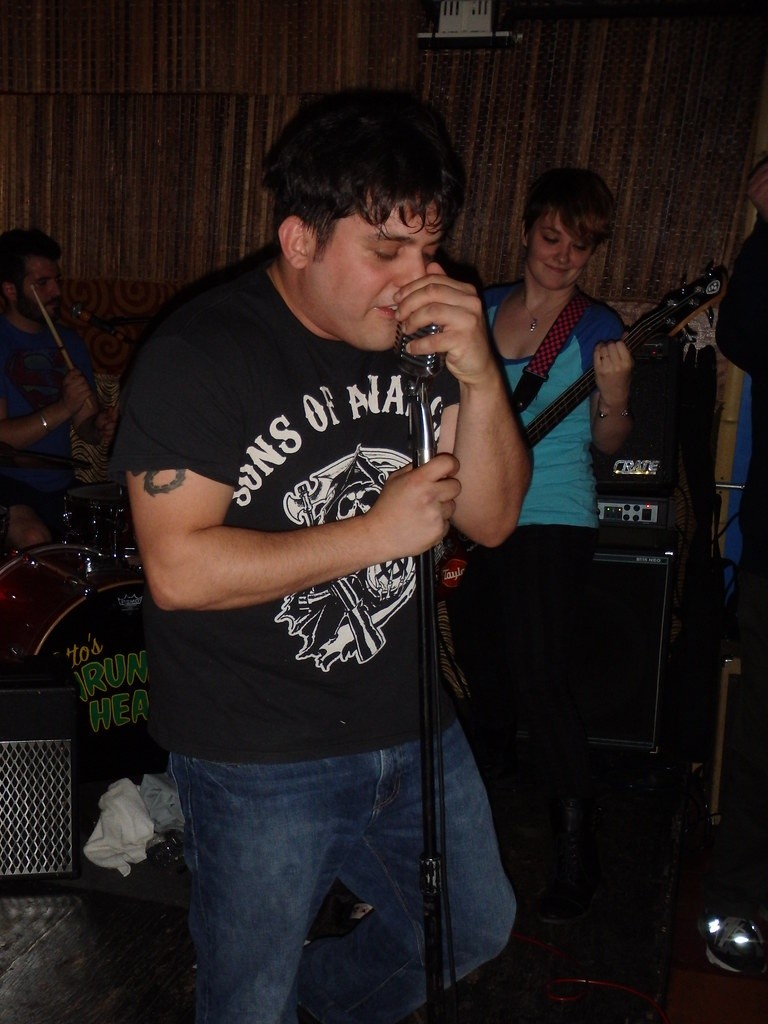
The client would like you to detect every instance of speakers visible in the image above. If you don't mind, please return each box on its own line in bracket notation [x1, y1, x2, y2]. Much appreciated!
[593, 336, 684, 498]
[514, 544, 676, 755]
[0, 654, 82, 883]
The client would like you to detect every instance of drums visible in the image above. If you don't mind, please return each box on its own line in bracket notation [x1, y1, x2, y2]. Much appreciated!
[1, 540, 145, 656]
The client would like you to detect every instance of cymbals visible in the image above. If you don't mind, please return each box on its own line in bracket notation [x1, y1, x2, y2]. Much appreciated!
[68, 481, 128, 501]
[0, 441, 92, 470]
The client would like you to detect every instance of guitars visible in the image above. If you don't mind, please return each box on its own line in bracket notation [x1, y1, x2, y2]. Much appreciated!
[435, 258, 729, 602]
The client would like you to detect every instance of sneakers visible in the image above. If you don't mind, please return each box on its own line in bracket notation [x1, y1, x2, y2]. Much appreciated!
[698, 905, 768, 975]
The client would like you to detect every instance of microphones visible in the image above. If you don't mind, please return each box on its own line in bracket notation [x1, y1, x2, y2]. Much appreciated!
[71, 302, 133, 345]
[391, 315, 446, 468]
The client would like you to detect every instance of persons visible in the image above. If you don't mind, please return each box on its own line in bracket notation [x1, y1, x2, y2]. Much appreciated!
[437, 165, 767, 977]
[0, 228, 95, 549]
[108, 89, 534, 1023]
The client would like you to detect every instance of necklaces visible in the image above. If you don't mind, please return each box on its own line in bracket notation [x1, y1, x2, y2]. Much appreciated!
[523, 291, 575, 332]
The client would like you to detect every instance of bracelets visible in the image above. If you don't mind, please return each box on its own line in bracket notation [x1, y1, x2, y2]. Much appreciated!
[597, 397, 630, 418]
[37, 411, 51, 434]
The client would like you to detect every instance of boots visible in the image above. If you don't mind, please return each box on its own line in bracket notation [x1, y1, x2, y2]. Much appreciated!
[536, 798, 600, 922]
